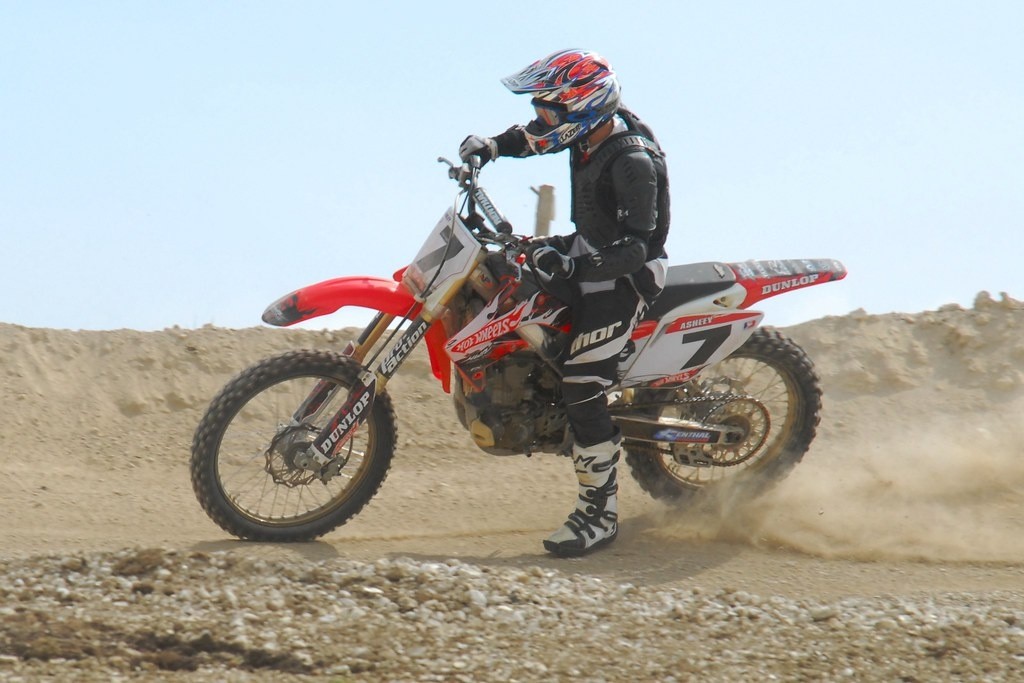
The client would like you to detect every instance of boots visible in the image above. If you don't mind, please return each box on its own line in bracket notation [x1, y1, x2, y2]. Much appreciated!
[543, 424, 622, 557]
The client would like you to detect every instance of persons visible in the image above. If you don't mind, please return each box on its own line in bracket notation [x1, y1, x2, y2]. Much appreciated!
[459, 48, 671, 556]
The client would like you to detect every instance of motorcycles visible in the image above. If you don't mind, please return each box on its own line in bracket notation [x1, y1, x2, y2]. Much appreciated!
[187, 145, 849, 544]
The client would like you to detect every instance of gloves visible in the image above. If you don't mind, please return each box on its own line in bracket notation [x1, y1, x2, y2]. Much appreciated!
[458, 133, 499, 162]
[532, 246, 575, 286]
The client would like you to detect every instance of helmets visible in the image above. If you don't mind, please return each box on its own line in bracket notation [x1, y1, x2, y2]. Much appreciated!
[499, 47, 623, 155]
